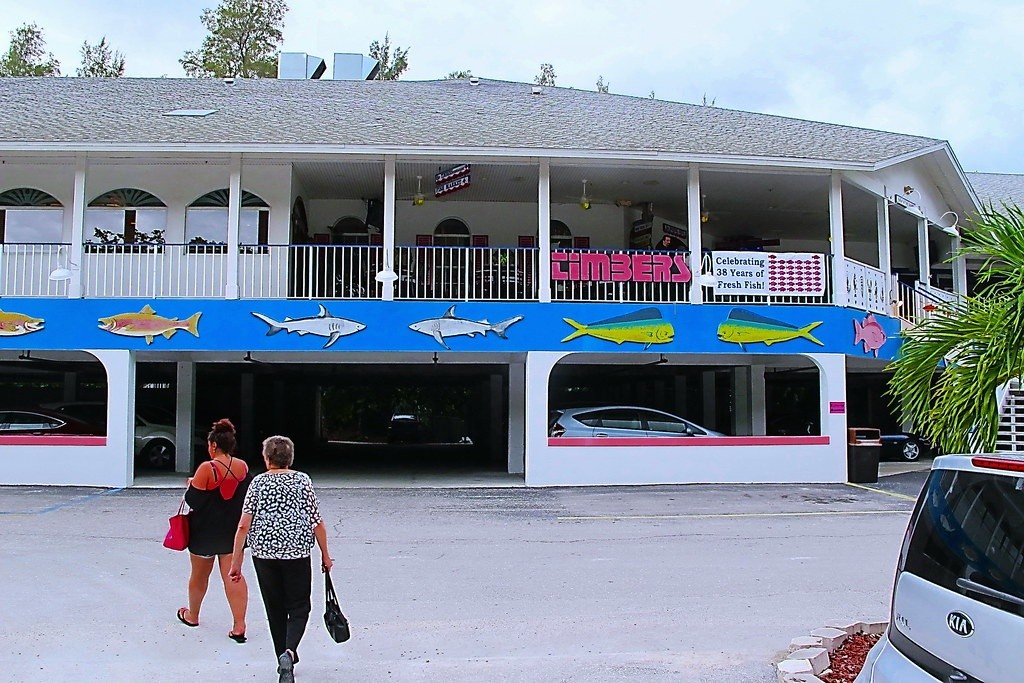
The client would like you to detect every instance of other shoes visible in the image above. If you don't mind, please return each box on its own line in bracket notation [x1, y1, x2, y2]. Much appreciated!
[279, 651, 295, 683]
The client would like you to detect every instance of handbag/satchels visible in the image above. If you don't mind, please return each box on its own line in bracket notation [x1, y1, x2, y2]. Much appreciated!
[162, 499, 190, 551]
[324, 567, 352, 643]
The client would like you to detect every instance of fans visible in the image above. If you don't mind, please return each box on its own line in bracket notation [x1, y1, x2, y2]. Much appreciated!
[401, 175, 435, 207]
[559, 179, 605, 209]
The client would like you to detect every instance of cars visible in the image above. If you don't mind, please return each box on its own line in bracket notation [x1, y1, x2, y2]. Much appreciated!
[37, 402, 208, 472]
[388, 412, 420, 445]
[548, 406, 729, 440]
[849, 446, 1024, 683]
[0, 403, 90, 435]
[878, 432, 927, 461]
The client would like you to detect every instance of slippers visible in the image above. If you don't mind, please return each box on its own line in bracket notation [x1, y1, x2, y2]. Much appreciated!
[229, 631, 245, 643]
[178, 609, 199, 626]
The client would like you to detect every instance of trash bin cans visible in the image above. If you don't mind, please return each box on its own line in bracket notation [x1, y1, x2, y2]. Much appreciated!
[847, 428, 883, 483]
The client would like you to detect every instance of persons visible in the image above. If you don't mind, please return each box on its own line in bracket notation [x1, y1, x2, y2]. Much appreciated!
[177, 418, 251, 644]
[227, 434, 333, 683]
[656, 234, 671, 250]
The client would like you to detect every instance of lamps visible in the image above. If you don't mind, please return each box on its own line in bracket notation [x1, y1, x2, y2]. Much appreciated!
[700, 254, 718, 288]
[890, 299, 905, 308]
[927, 211, 962, 238]
[701, 194, 728, 222]
[887, 187, 924, 219]
[49, 245, 79, 281]
[374, 248, 396, 281]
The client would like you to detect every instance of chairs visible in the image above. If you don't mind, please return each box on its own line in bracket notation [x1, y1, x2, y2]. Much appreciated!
[330, 266, 365, 296]
[395, 262, 429, 296]
